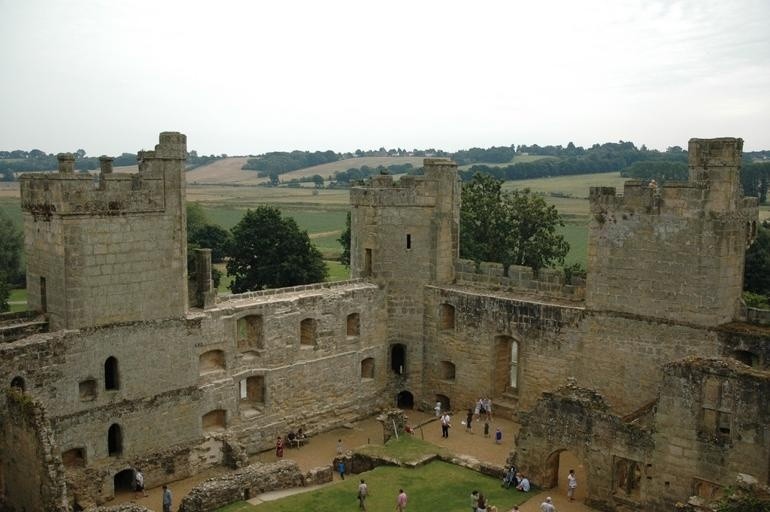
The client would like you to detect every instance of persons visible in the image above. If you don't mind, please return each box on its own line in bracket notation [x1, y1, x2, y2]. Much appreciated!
[336, 439, 344, 455]
[134, 468, 149, 499]
[275, 436, 284, 458]
[433, 395, 502, 445]
[470, 465, 577, 512]
[357, 478, 370, 512]
[338, 461, 346, 480]
[405, 422, 413, 433]
[395, 488, 408, 512]
[161, 484, 172, 512]
[232, 446, 249, 467]
